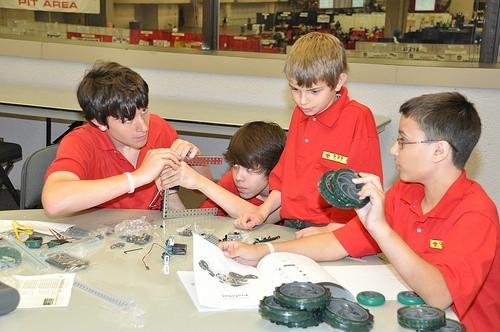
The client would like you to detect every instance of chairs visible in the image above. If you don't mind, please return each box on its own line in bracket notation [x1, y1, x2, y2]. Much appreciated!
[0, 137, 22, 207]
[21, 144, 61, 209]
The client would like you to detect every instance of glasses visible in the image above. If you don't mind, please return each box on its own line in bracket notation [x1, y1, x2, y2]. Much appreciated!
[398, 137, 457, 153]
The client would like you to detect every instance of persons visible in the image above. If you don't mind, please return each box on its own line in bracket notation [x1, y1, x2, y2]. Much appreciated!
[233, 32, 384, 240]
[154, 118, 287, 226]
[41, 59, 202, 217]
[218, 90, 500, 332]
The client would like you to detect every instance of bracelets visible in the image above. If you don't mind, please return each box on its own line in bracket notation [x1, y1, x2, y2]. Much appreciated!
[263, 243, 274, 253]
[169, 191, 178, 195]
[123, 172, 135, 193]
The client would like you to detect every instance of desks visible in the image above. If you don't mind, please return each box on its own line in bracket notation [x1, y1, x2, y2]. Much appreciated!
[0, 209, 461, 332]
